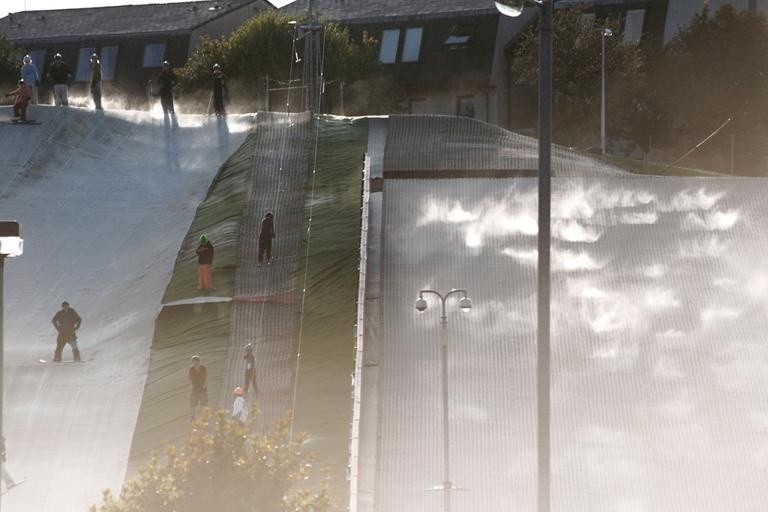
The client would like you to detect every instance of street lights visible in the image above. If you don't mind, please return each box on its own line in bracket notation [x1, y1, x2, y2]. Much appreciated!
[495, 0, 551, 510]
[597, 16, 612, 157]
[1, 219, 22, 476]
[417, 287, 471, 507]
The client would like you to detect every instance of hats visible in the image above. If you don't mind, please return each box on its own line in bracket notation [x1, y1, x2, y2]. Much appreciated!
[244, 343, 252, 351]
[234, 387, 243, 394]
[200, 234, 208, 244]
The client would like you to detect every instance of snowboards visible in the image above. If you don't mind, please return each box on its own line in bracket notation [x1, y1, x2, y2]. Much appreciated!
[0, 118, 42, 125]
[40, 359, 93, 363]
[1, 477, 27, 499]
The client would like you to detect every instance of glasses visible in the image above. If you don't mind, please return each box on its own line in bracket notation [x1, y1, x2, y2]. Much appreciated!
[163, 61, 169, 64]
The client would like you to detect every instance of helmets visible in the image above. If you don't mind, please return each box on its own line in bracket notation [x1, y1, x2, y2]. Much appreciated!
[23, 55, 32, 61]
[90, 54, 99, 59]
[54, 53, 62, 60]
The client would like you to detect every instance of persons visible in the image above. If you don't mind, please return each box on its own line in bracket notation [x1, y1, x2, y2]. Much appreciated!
[154, 61, 180, 115]
[242, 342, 259, 401]
[4, 80, 32, 123]
[209, 62, 229, 118]
[232, 387, 249, 426]
[51, 299, 84, 362]
[257, 208, 275, 270]
[1, 434, 16, 491]
[20, 54, 41, 106]
[194, 234, 217, 293]
[88, 52, 104, 110]
[186, 354, 208, 421]
[46, 52, 74, 108]
